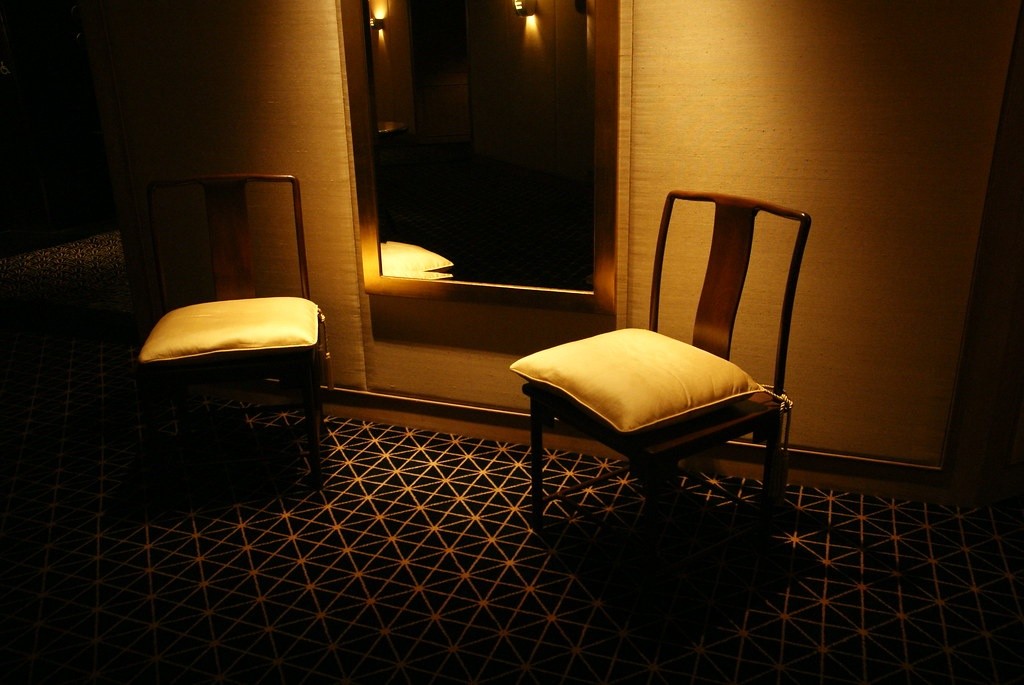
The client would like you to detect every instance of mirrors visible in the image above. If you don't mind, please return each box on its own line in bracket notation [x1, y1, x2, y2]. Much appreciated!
[341, 0, 617, 315]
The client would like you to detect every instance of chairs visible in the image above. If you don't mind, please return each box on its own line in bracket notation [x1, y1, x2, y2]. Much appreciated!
[510, 191, 811, 585]
[145, 174, 327, 487]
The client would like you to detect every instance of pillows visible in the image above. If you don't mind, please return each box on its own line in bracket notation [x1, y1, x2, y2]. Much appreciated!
[138, 297, 319, 367]
[509, 326, 763, 433]
